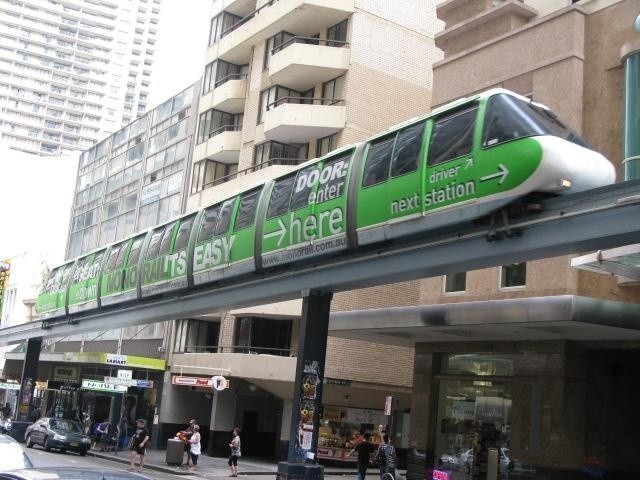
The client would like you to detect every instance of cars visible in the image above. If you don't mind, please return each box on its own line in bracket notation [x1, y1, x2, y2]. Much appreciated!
[24, 416, 92, 456]
[414, 447, 459, 471]
[459, 447, 537, 478]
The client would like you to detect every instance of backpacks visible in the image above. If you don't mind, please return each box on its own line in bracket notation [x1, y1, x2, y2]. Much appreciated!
[376, 446, 388, 468]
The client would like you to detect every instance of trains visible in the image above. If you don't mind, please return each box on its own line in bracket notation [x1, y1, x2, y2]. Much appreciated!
[35, 88, 617, 325]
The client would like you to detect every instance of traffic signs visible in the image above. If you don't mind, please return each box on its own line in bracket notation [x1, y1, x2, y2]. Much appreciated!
[104, 376, 153, 388]
[82, 379, 124, 393]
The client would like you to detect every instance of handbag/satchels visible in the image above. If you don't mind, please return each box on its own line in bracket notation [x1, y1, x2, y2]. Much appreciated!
[127, 434, 136, 450]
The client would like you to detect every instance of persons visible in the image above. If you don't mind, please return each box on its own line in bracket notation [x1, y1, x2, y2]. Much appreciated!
[127, 420, 151, 474]
[190, 427, 202, 468]
[92, 416, 128, 451]
[347, 433, 373, 479]
[376, 435, 399, 478]
[181, 420, 197, 465]
[227, 427, 242, 478]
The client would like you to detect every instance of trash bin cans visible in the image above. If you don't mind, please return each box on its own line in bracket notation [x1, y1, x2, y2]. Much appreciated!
[166, 439, 184, 467]
